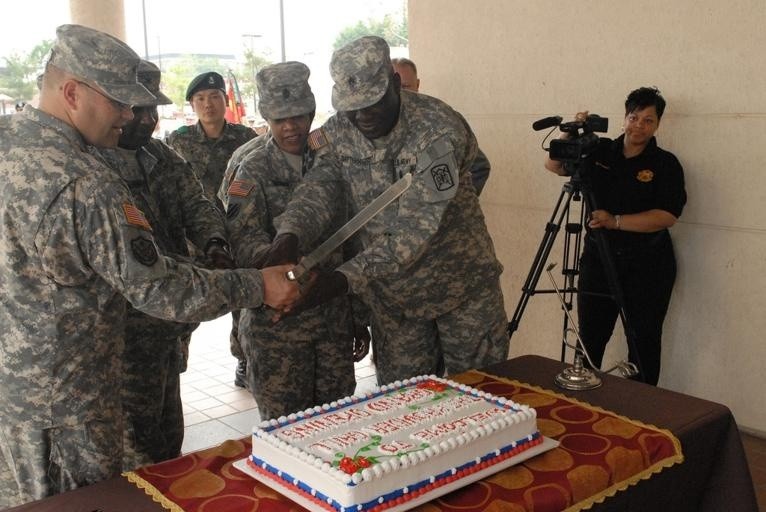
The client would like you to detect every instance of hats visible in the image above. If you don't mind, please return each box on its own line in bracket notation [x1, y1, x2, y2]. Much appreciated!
[257, 63, 315, 120]
[330, 35, 392, 111]
[185, 73, 224, 101]
[48, 24, 173, 106]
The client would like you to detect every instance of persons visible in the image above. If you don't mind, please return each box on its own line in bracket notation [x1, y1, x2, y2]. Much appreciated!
[256, 36, 509, 384]
[215, 61, 370, 422]
[1, 24, 315, 508]
[98, 60, 231, 474]
[163, 72, 259, 392]
[544, 87, 687, 387]
[391, 57, 419, 92]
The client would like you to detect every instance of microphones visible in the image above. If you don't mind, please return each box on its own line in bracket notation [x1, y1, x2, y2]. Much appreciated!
[533, 115, 561, 131]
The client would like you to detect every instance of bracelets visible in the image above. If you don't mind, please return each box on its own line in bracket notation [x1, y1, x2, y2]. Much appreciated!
[615, 214, 620, 230]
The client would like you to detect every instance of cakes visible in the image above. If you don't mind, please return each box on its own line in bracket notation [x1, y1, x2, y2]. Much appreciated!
[247, 374, 545, 511]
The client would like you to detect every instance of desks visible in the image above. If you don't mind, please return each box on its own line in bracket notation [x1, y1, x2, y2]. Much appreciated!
[0, 353, 766, 511]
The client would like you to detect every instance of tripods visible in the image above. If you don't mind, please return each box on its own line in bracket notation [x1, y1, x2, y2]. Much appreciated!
[507, 180, 646, 383]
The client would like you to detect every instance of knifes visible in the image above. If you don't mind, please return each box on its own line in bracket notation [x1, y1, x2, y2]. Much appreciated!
[288, 172, 413, 282]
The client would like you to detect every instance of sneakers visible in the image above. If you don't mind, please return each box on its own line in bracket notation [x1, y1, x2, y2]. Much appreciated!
[235, 357, 249, 387]
